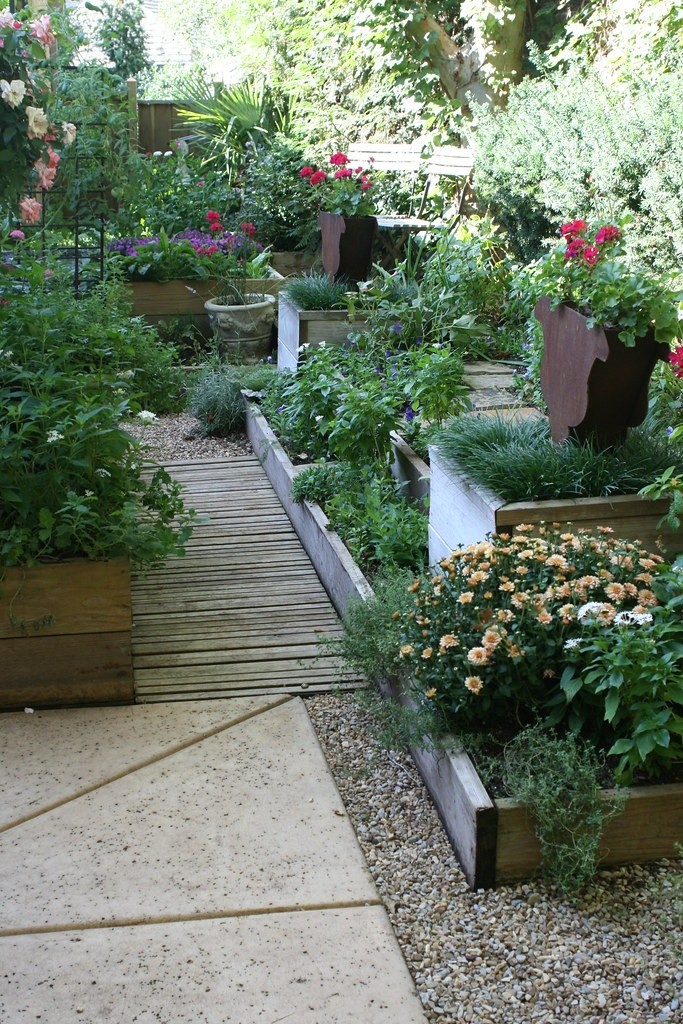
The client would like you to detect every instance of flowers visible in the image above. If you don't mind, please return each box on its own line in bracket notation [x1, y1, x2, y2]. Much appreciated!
[104, 210, 276, 281]
[298, 150, 383, 218]
[524, 215, 683, 381]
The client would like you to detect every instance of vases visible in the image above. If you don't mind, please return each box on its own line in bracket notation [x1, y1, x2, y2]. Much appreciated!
[104, 266, 281, 359]
[385, 342, 474, 517]
[529, 295, 669, 449]
[204, 242, 276, 364]
[318, 211, 378, 285]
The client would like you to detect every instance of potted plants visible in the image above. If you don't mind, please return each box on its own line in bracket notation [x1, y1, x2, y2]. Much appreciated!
[277, 266, 433, 376]
[426, 407, 683, 601]
[1, 240, 209, 711]
